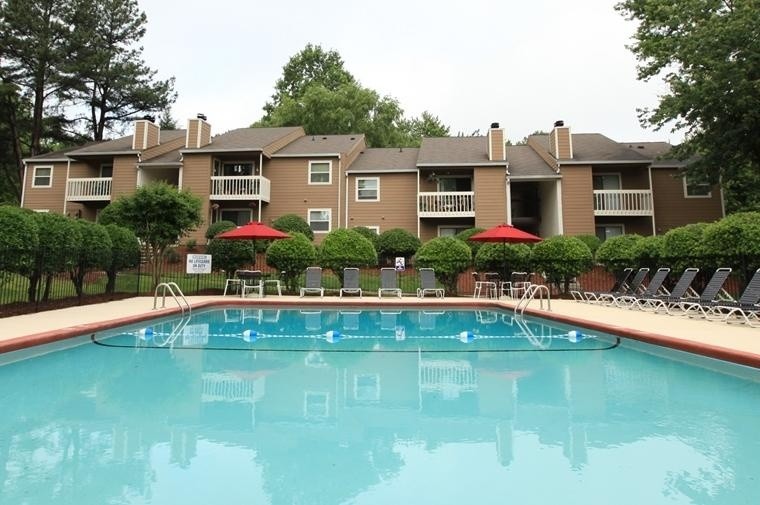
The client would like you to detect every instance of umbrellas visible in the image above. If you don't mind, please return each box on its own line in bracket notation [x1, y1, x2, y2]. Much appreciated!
[467, 222, 544, 295]
[214, 220, 292, 291]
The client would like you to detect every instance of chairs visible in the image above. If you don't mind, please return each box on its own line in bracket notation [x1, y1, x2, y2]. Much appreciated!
[298, 266, 445, 299]
[470, 269, 534, 300]
[223, 308, 280, 325]
[297, 309, 444, 331]
[569, 268, 760, 329]
[222, 270, 286, 298]
